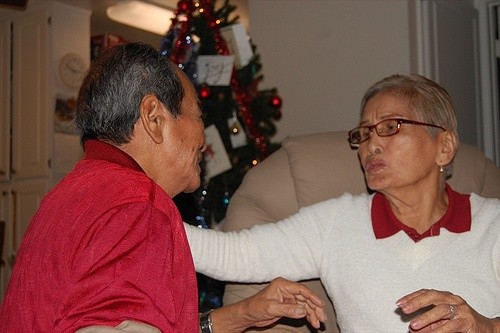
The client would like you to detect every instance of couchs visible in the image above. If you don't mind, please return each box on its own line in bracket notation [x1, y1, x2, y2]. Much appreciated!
[221, 130, 500, 333]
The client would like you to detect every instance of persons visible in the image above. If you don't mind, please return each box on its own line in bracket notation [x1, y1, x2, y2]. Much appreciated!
[182, 75, 500, 333]
[0, 42, 328, 333]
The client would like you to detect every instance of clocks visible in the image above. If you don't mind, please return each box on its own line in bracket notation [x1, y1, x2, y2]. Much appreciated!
[59, 52, 88, 89]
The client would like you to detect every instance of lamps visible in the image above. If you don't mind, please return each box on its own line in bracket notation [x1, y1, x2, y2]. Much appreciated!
[106, 1, 176, 37]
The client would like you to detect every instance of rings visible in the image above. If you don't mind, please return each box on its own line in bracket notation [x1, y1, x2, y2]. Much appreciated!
[447, 304, 455, 318]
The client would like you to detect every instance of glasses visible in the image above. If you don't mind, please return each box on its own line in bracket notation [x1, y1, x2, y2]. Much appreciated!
[348, 118, 445, 143]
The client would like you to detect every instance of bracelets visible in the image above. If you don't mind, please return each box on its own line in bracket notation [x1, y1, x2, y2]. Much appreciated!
[200, 309, 215, 333]
